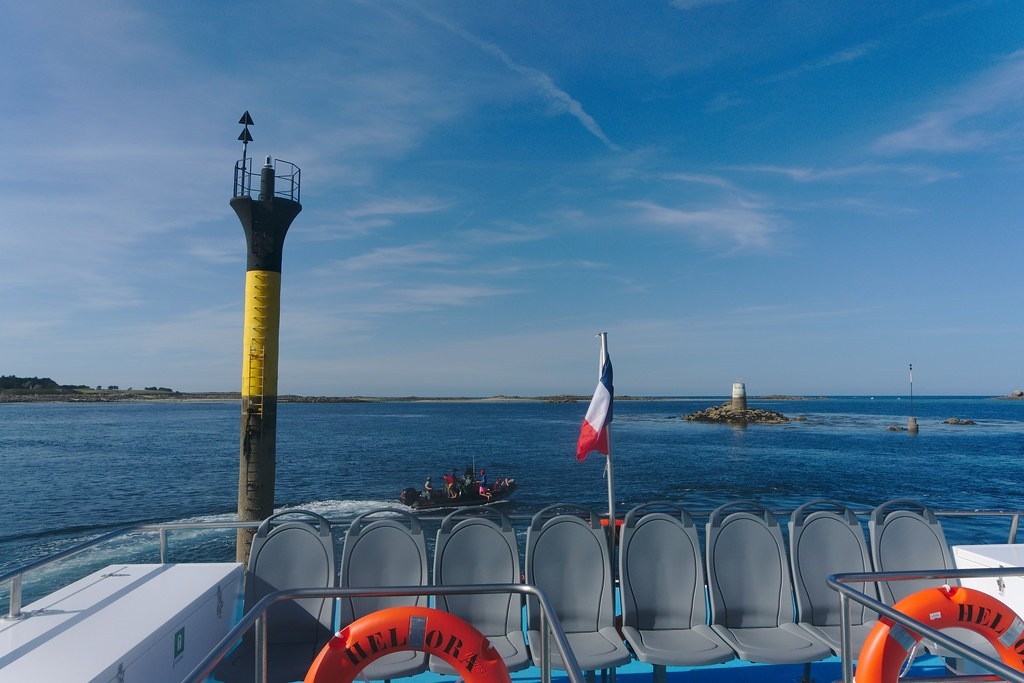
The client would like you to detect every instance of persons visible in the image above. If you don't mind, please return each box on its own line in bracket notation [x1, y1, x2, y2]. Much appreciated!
[475, 470, 492, 500]
[424, 477, 432, 501]
[494, 477, 509, 493]
[443, 468, 463, 499]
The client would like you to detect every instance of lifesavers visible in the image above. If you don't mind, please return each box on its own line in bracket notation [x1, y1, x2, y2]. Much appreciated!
[854, 582, 1024, 683]
[587, 518, 625, 544]
[304, 605, 512, 683]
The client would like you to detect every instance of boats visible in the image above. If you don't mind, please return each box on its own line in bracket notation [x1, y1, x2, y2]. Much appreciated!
[397, 455, 518, 511]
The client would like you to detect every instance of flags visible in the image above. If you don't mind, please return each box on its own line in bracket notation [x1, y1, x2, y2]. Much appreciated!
[575, 347, 614, 464]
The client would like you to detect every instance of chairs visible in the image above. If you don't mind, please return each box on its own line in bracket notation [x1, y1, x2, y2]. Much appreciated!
[214, 500, 957, 683]
